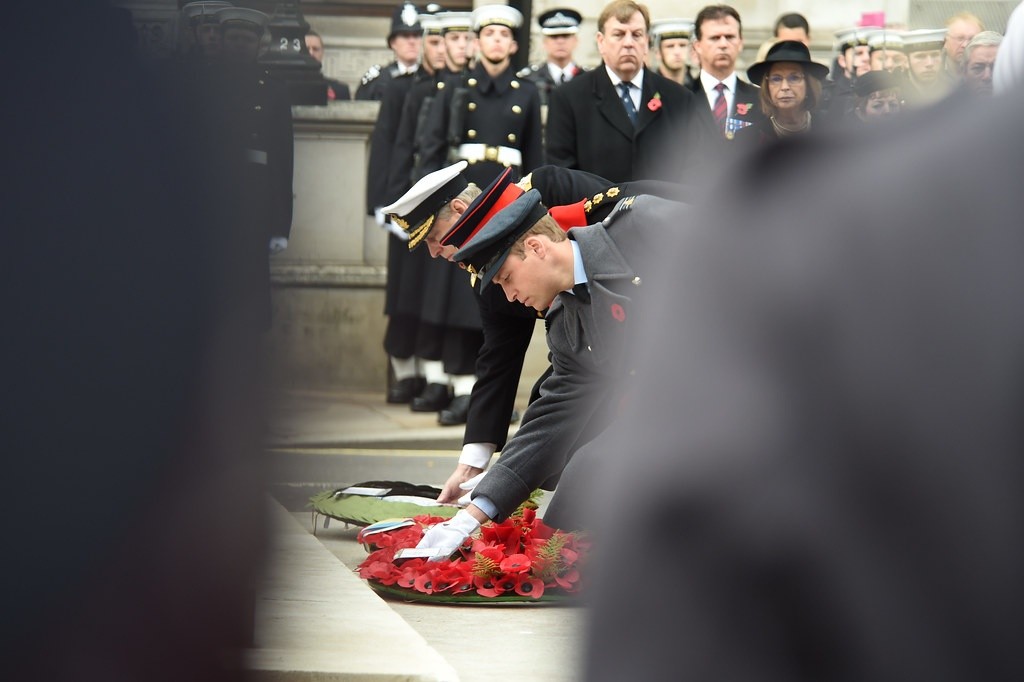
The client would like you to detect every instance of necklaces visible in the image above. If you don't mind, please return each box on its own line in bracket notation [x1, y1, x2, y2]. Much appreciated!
[770, 111, 812, 137]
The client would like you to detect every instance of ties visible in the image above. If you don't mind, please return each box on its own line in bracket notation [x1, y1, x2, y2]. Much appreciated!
[712, 83, 728, 139]
[572, 284, 591, 305]
[617, 80, 638, 128]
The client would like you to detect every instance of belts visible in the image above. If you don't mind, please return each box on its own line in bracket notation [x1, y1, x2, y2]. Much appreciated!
[445, 143, 523, 167]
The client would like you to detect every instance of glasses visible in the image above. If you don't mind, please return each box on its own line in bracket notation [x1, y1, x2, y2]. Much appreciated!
[765, 72, 806, 85]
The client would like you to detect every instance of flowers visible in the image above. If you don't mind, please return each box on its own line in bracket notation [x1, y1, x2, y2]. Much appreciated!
[355, 506, 586, 596]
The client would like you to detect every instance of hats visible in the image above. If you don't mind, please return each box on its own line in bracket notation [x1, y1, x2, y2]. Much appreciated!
[747, 40, 829, 86]
[853, 70, 900, 97]
[833, 25, 949, 52]
[435, 11, 473, 36]
[386, 3, 425, 49]
[182, 0, 235, 24]
[470, 4, 524, 32]
[538, 8, 582, 36]
[418, 14, 443, 34]
[452, 188, 548, 295]
[649, 17, 697, 40]
[438, 167, 526, 249]
[380, 160, 468, 254]
[213, 6, 270, 37]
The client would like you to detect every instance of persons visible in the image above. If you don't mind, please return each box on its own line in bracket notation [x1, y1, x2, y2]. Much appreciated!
[119, 0, 1024, 682]
[542, 1, 713, 196]
[684, 3, 767, 165]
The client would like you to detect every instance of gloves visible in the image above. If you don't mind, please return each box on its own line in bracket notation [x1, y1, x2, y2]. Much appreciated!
[457, 471, 487, 506]
[393, 508, 481, 562]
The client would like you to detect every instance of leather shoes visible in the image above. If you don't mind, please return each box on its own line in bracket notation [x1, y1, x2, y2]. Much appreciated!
[438, 395, 471, 424]
[387, 377, 426, 403]
[411, 383, 448, 411]
